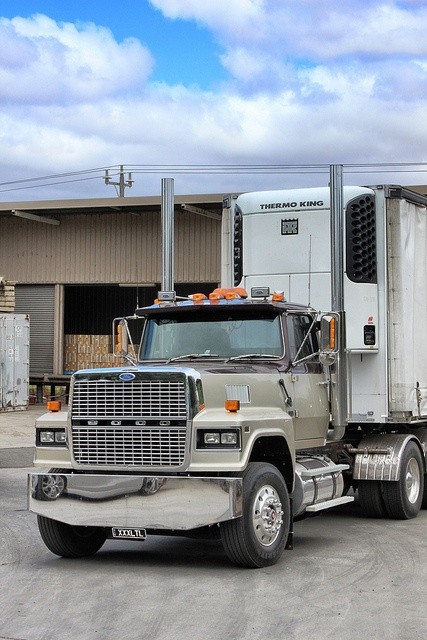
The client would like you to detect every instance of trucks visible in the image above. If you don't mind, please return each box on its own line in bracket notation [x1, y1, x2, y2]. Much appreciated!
[28, 182, 426, 571]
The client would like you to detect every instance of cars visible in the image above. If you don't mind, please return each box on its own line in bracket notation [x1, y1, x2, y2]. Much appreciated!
[29, 475, 166, 502]
